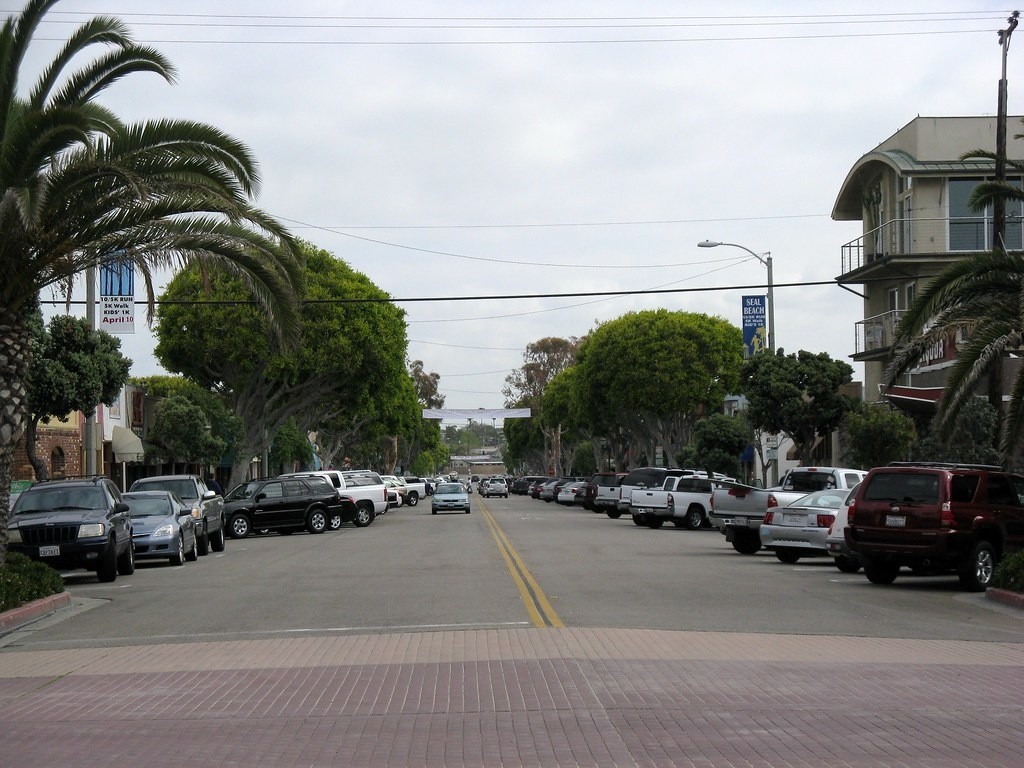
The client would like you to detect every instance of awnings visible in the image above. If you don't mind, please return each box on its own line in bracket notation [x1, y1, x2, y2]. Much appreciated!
[738, 444, 754, 460]
[112, 426, 144, 463]
[786, 436, 825, 460]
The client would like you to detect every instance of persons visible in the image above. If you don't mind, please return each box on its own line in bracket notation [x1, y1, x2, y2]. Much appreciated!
[205, 473, 223, 496]
[467, 478, 472, 491]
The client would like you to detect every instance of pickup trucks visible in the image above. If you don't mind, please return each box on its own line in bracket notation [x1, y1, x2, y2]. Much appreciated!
[708, 466, 885, 555]
[129, 473, 226, 555]
[629, 475, 739, 530]
[342, 470, 459, 527]
[279, 470, 389, 527]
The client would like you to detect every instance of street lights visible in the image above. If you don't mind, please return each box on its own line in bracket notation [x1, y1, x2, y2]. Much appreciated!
[698, 239, 778, 487]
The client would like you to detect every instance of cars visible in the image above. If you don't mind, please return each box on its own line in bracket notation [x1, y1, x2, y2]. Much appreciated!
[120, 490, 198, 565]
[430, 483, 470, 514]
[508, 476, 591, 510]
[759, 480, 863, 572]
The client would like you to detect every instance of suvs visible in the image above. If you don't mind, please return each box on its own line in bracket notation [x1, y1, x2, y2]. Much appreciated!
[584, 472, 629, 519]
[844, 461, 1024, 592]
[471, 476, 479, 483]
[478, 476, 509, 498]
[224, 476, 342, 539]
[8, 474, 134, 583]
[616, 466, 726, 526]
